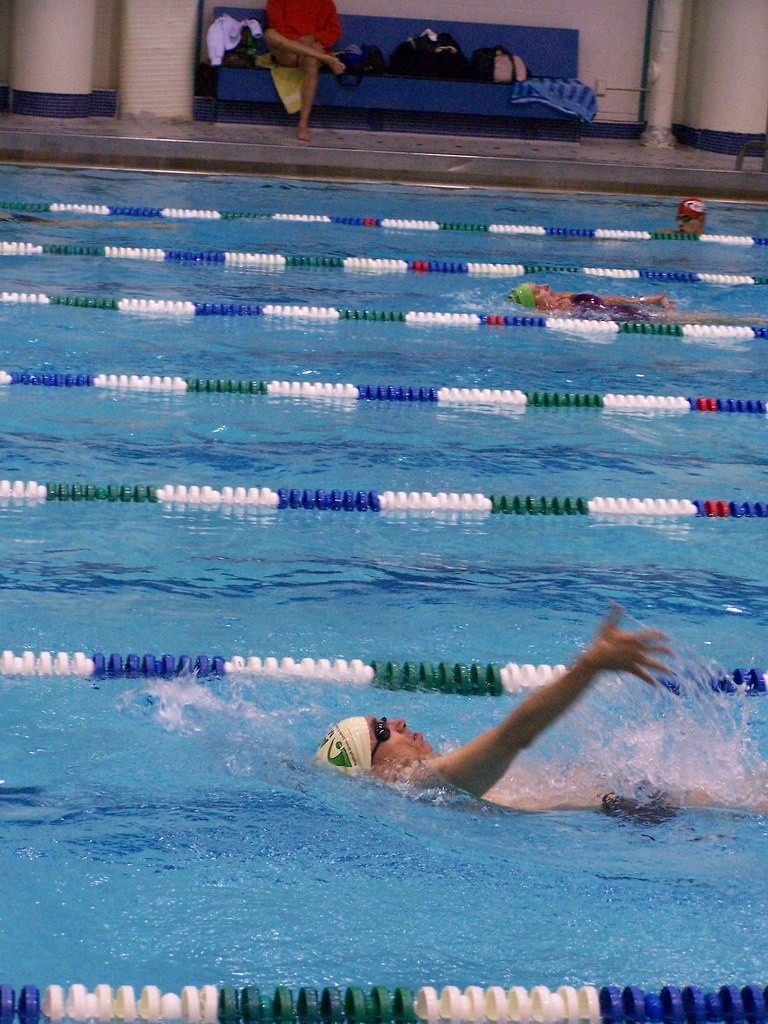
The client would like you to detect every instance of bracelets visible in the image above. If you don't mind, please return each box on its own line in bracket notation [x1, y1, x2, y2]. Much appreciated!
[639, 296, 645, 302]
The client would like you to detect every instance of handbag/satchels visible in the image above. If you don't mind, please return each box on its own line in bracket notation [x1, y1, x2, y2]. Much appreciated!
[388, 29, 469, 82]
[472, 45, 528, 83]
[222, 26, 258, 69]
[358, 44, 386, 76]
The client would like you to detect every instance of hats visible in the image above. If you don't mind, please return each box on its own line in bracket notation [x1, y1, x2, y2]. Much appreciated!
[676, 198, 706, 221]
[315, 716, 371, 778]
[505, 282, 534, 311]
[345, 45, 362, 55]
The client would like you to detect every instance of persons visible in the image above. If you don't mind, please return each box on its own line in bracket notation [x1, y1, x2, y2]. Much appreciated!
[658, 198, 707, 234]
[315, 601, 674, 826]
[264, 0, 346, 142]
[505, 282, 679, 323]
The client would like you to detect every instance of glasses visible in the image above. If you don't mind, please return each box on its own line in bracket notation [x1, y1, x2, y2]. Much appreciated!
[675, 215, 693, 223]
[371, 716, 391, 764]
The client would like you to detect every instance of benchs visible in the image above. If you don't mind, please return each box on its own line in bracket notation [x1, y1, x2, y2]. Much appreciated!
[212, 6, 598, 140]
